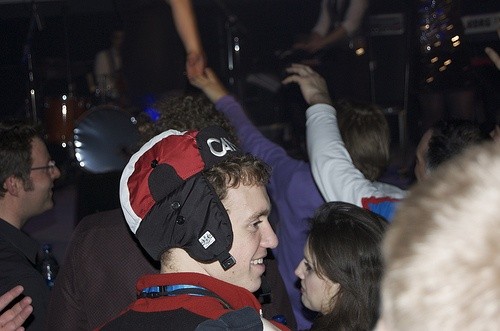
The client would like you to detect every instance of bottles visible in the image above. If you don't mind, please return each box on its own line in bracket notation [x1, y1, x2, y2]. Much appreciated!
[39, 244, 59, 289]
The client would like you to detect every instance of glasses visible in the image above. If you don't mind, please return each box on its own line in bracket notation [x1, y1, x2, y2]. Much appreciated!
[29, 161, 55, 171]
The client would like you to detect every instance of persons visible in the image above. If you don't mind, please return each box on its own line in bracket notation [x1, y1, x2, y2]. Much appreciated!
[95, 28, 134, 108]
[170, 1, 208, 83]
[91, 126, 292, 331]
[284, 0, 372, 107]
[295, 200, 391, 331]
[375, 130, 500, 331]
[190, 68, 393, 331]
[0, 125, 62, 331]
[283, 63, 495, 222]
[45, 86, 237, 331]
[0, 285, 33, 331]
[406, 0, 476, 134]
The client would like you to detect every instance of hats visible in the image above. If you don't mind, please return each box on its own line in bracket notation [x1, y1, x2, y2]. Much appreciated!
[119, 126, 243, 271]
